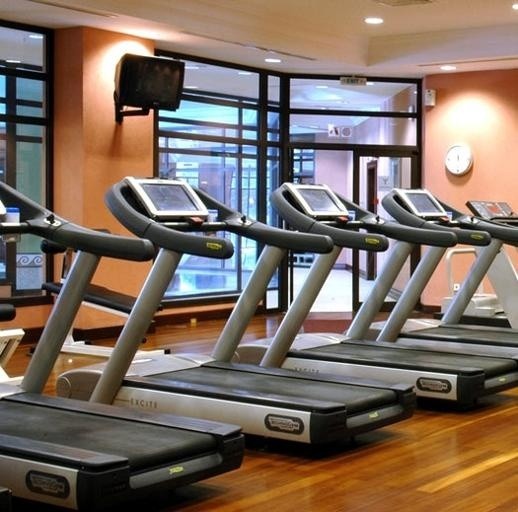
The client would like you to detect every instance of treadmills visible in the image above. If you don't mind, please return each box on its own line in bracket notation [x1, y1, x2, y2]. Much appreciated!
[344, 188, 518, 363]
[1, 180, 244, 511]
[57, 176, 418, 452]
[228, 183, 518, 406]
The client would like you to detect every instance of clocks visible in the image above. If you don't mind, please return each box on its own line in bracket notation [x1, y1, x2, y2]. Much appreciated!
[444, 144, 474, 176]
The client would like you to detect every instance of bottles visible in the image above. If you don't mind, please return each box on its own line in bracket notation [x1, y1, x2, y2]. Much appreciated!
[207, 209, 219, 236]
[348, 211, 356, 228]
[3, 207, 24, 242]
[446, 212, 452, 224]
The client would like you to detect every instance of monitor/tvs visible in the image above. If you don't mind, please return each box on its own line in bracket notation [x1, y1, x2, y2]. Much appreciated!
[287, 181, 349, 219]
[113, 53, 187, 113]
[395, 189, 447, 221]
[128, 174, 208, 218]
